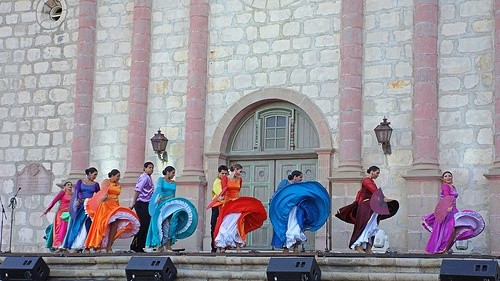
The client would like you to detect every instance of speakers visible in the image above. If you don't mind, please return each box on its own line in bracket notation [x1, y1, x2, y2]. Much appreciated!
[266, 257, 321, 281]
[125, 256, 177, 281]
[0, 257, 50, 281]
[440, 259, 500, 281]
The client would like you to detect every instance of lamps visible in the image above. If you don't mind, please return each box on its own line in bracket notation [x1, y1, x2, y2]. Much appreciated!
[374, 115, 393, 155]
[149, 126, 168, 163]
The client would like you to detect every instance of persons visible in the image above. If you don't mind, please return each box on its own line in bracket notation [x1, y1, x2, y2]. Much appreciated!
[335, 165, 399, 254]
[128, 162, 153, 253]
[143, 166, 198, 252]
[421, 171, 485, 255]
[83, 169, 141, 254]
[40, 181, 74, 252]
[210, 165, 228, 252]
[59, 167, 101, 254]
[206, 164, 267, 252]
[268, 170, 332, 252]
